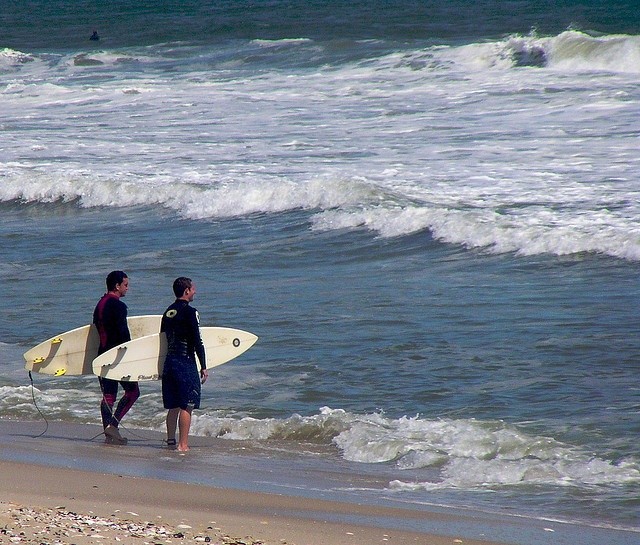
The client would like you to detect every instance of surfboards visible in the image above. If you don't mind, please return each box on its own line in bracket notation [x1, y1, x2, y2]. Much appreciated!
[90, 325, 259, 382]
[21, 314, 167, 377]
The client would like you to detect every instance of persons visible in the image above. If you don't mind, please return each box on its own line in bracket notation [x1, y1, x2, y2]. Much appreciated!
[93, 271, 140, 445]
[161, 277, 208, 452]
